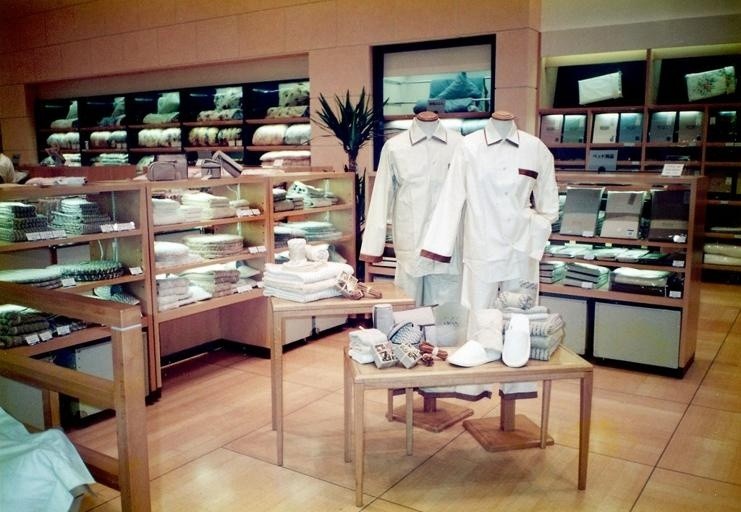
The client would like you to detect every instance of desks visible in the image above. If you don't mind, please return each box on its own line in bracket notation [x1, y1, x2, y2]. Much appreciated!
[342, 343, 594, 509]
[267, 278, 415, 466]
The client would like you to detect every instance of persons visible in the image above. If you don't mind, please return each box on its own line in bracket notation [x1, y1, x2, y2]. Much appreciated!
[423, 112, 559, 309]
[358, 111, 465, 309]
[0, 128, 15, 183]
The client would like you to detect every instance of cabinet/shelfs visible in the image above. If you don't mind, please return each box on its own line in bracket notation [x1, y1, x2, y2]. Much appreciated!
[146, 170, 357, 400]
[0, 179, 159, 430]
[362, 32, 739, 379]
[23, 52, 310, 178]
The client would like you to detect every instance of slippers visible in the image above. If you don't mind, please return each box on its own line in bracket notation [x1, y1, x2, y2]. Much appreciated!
[446, 340, 502, 367]
[501, 314, 531, 367]
